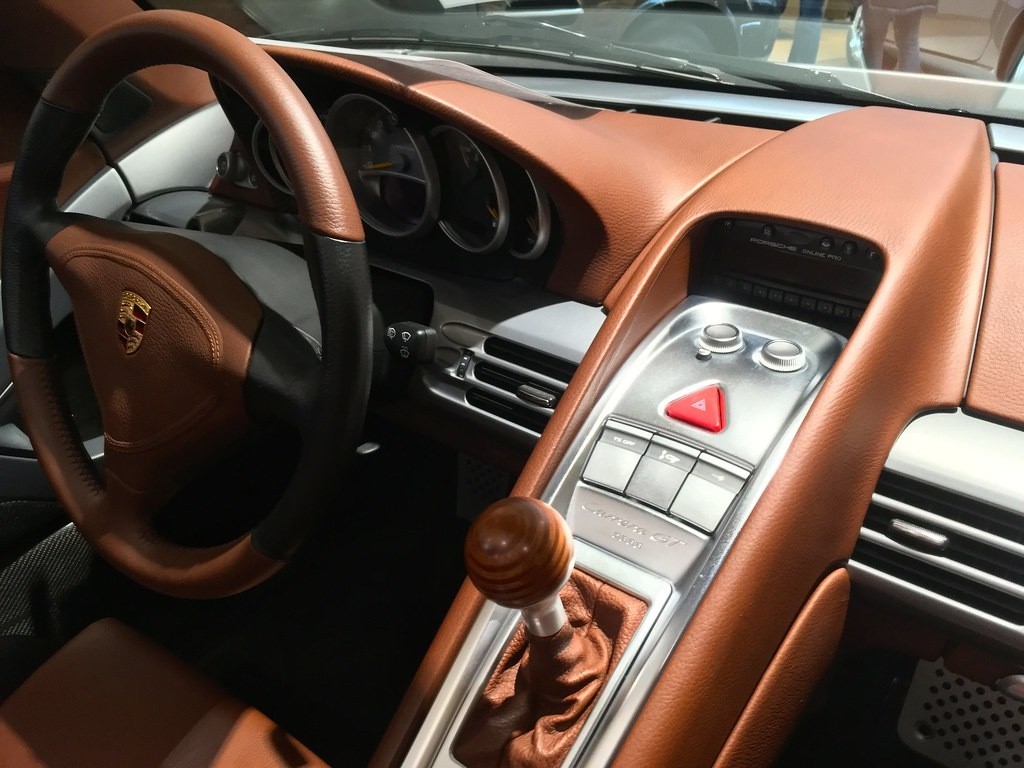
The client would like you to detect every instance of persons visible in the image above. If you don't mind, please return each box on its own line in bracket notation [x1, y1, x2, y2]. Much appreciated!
[763, 0, 827, 64]
[862, 0, 938, 74]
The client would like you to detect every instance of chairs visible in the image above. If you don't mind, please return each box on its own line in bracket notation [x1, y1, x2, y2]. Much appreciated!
[0, 617, 333, 768]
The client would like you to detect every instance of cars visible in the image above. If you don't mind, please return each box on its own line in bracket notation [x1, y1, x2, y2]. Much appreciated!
[382, 0, 790, 59]
[844, 1, 1024, 83]
